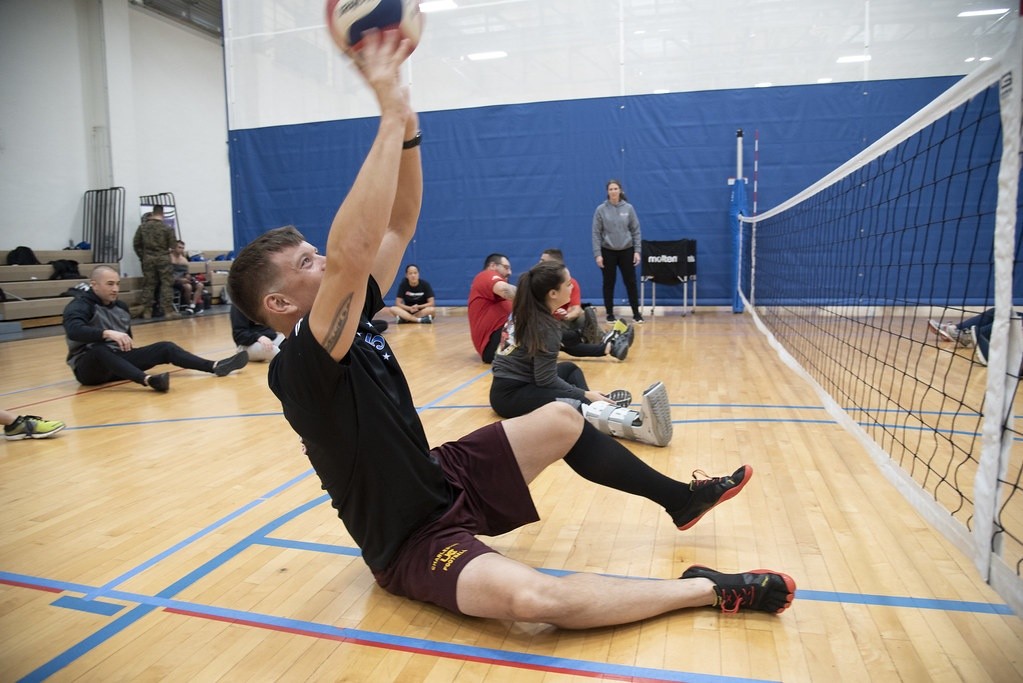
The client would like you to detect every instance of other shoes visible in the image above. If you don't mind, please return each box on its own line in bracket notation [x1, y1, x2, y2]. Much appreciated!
[141, 307, 154, 319]
[185, 306, 194, 314]
[603, 390, 631, 406]
[194, 307, 203, 313]
[164, 309, 183, 320]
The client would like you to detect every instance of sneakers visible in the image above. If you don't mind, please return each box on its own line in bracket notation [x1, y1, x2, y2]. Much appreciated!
[602, 329, 620, 346]
[667, 464, 752, 531]
[396, 315, 406, 324]
[4, 413, 66, 441]
[213, 351, 248, 376]
[420, 314, 433, 324]
[678, 565, 797, 614]
[946, 327, 971, 347]
[633, 314, 645, 324]
[926, 319, 955, 342]
[606, 314, 617, 323]
[147, 372, 170, 391]
[971, 324, 990, 366]
[610, 324, 635, 360]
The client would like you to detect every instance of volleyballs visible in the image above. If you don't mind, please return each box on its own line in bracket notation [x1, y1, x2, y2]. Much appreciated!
[326, 0, 424, 66]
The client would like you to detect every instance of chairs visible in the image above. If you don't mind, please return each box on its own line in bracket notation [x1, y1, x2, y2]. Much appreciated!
[639, 239, 696, 317]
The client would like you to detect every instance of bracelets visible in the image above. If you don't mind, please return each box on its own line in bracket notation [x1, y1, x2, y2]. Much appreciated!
[402, 132, 422, 150]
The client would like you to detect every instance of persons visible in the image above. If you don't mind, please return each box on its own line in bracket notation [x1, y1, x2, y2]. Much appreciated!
[539, 249, 584, 323]
[489, 261, 673, 445]
[390, 264, 437, 324]
[0, 410, 66, 440]
[168, 241, 203, 314]
[132, 205, 182, 319]
[467, 253, 634, 364]
[591, 180, 645, 324]
[62, 265, 248, 392]
[928, 307, 995, 366]
[226, 28, 796, 629]
[229, 304, 285, 362]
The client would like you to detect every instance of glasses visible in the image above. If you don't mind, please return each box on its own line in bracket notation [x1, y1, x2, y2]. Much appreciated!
[496, 263, 512, 273]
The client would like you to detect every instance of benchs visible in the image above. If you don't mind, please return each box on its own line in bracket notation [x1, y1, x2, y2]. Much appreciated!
[0, 249, 235, 334]
